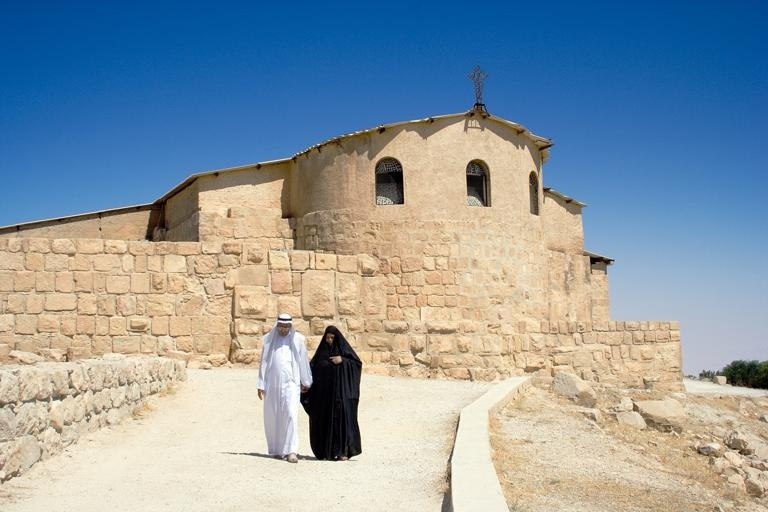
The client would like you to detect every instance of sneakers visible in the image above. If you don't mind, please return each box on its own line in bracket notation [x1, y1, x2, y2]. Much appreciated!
[287, 453, 298, 463]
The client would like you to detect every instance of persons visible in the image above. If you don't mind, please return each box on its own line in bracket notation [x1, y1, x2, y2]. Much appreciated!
[302, 326, 362, 461]
[258, 314, 314, 463]
